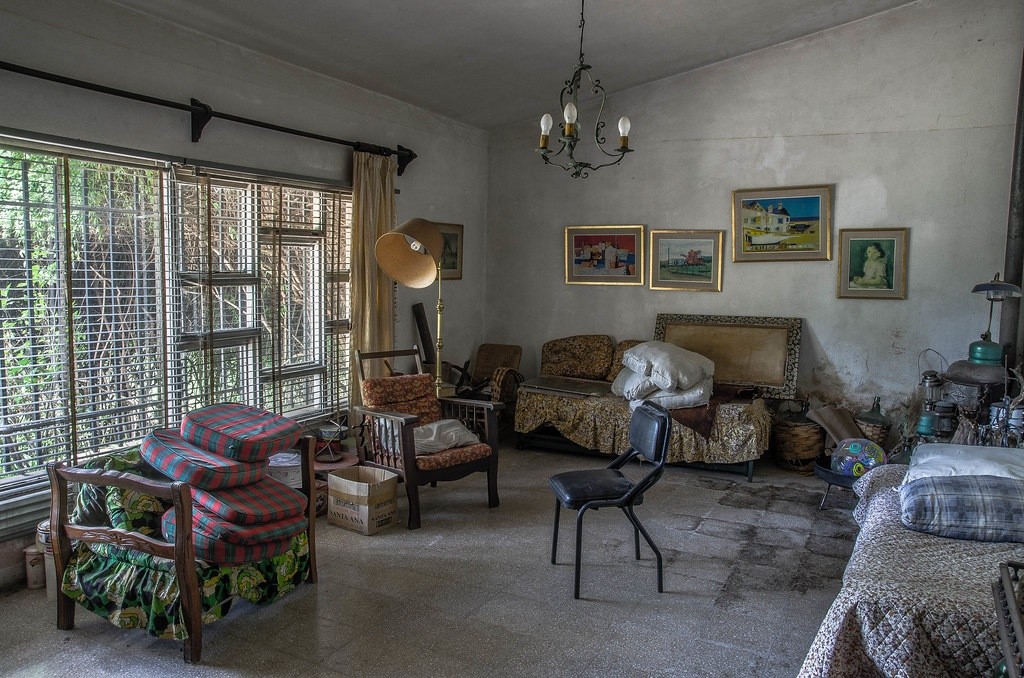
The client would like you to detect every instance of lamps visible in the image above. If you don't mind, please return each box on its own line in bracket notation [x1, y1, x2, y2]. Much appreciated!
[533, 0, 634, 180]
[374, 218, 446, 402]
[968, 272, 1024, 368]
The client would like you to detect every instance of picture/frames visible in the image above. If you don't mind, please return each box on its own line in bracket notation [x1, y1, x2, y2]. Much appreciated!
[655, 313, 802, 400]
[564, 225, 646, 287]
[731, 184, 833, 262]
[434, 222, 465, 280]
[838, 227, 907, 301]
[649, 229, 724, 294]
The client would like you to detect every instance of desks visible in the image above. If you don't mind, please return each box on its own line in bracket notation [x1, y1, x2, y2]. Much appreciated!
[797, 463, 1024, 678]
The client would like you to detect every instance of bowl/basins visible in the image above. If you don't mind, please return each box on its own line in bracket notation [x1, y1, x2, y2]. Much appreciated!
[813, 455, 863, 488]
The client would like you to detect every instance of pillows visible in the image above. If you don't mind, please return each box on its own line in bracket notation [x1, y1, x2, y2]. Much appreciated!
[68, 447, 173, 540]
[374, 419, 480, 458]
[611, 340, 715, 414]
[898, 441, 1024, 544]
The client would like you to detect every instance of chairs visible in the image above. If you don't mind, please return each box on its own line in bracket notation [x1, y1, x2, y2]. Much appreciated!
[460, 341, 526, 439]
[47, 437, 318, 667]
[544, 400, 672, 600]
[353, 345, 500, 530]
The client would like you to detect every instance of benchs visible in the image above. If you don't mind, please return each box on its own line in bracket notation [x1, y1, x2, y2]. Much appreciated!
[515, 333, 770, 482]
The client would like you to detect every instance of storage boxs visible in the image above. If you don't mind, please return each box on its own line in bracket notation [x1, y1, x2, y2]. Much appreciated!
[327, 465, 399, 536]
